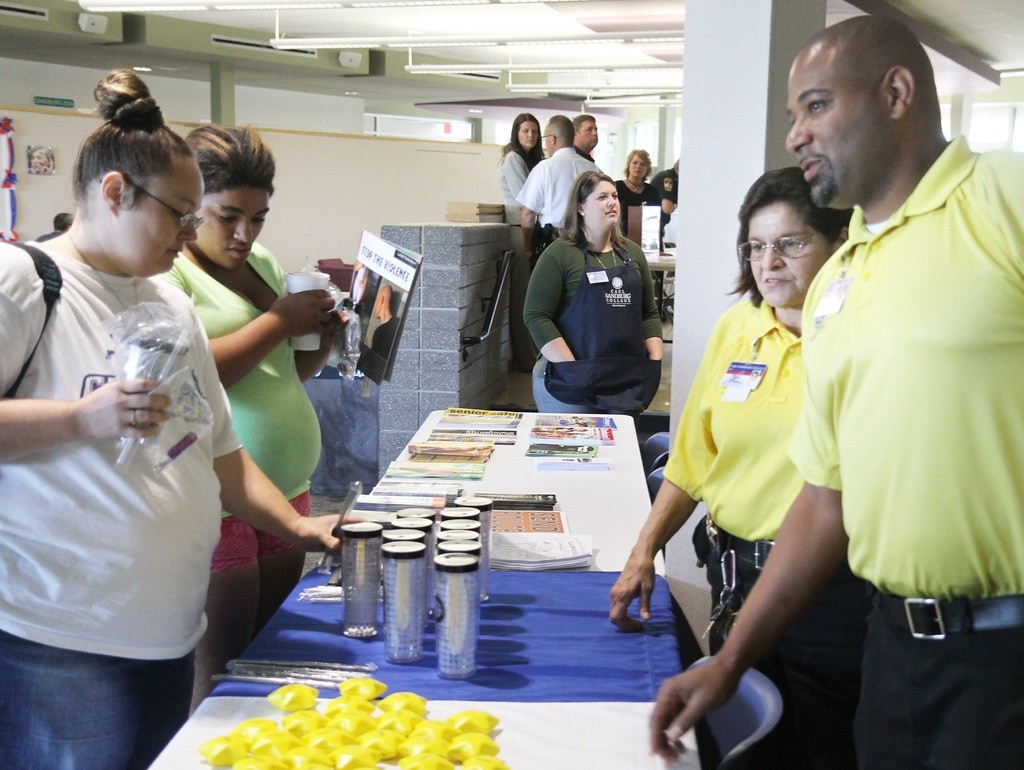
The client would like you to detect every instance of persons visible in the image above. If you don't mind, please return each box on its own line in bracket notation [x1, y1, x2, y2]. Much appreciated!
[650, 157, 680, 300]
[371, 285, 401, 358]
[36, 212, 73, 242]
[30, 146, 55, 174]
[610, 167, 853, 769]
[155, 124, 343, 718]
[614, 149, 660, 238]
[0, 68, 342, 770]
[497, 113, 599, 372]
[523, 171, 663, 429]
[649, 15, 1024, 769]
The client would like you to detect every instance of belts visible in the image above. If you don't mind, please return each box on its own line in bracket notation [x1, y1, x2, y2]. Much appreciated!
[719, 532, 775, 570]
[880, 594, 1024, 642]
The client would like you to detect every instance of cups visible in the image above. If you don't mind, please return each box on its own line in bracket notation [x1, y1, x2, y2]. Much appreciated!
[286, 272, 330, 350]
[125, 338, 189, 387]
[341, 495, 494, 679]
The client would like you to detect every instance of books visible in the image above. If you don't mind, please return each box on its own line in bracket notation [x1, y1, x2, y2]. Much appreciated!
[384, 406, 617, 482]
[344, 233, 424, 387]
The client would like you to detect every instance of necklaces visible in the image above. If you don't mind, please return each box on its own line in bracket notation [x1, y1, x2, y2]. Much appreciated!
[628, 179, 642, 191]
[69, 232, 139, 310]
[593, 247, 617, 269]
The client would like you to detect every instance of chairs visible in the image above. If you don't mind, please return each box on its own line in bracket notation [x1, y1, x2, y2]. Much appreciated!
[679, 655, 783, 770]
[641, 432, 670, 507]
[650, 271, 674, 326]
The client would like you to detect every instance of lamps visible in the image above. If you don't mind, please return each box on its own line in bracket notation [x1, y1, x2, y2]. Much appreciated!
[80, 0, 686, 109]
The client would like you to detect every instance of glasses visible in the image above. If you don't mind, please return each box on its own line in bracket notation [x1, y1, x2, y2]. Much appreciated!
[98, 170, 205, 232]
[736, 231, 824, 261]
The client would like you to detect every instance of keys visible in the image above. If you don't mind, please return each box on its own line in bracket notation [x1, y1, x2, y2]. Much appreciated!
[700, 599, 736, 640]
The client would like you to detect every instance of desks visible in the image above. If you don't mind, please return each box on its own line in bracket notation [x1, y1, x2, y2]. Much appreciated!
[148, 409, 702, 770]
[644, 247, 676, 319]
